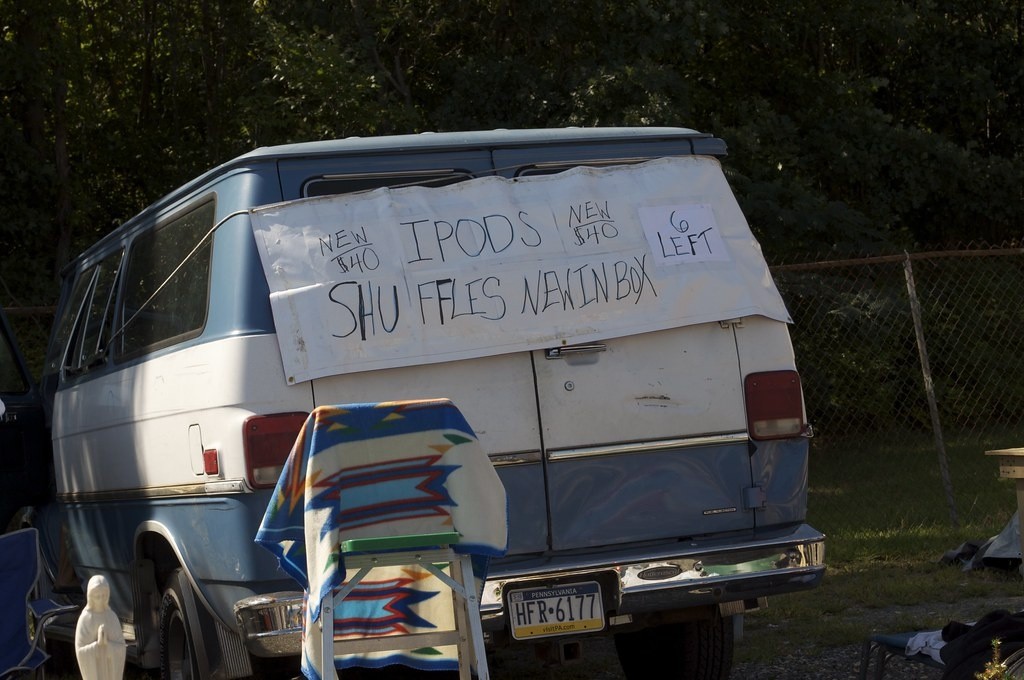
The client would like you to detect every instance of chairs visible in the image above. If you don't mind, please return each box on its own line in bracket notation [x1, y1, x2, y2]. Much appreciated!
[858, 609, 1024, 680]
[0, 528, 80, 680]
[254, 394, 509, 680]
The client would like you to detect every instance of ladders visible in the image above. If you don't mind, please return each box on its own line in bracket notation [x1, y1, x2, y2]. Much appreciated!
[303, 400, 499, 680]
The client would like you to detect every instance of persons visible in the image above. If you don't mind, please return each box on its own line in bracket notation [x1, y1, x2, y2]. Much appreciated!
[75, 575, 126, 680]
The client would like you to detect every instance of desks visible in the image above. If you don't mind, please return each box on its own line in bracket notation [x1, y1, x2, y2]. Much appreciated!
[984, 447, 1024, 567]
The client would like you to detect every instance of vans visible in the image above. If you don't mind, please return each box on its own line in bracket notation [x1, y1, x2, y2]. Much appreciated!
[0, 127, 829, 680]
